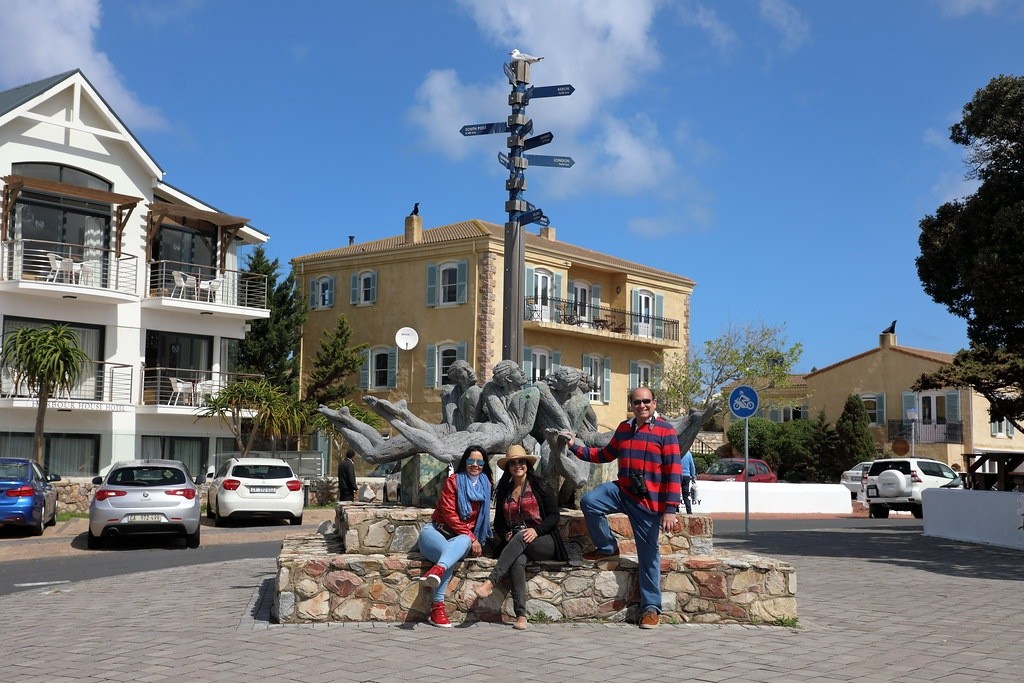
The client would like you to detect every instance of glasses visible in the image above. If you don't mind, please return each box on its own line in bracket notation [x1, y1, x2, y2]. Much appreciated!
[507, 459, 527, 467]
[465, 458, 485, 467]
[631, 398, 655, 405]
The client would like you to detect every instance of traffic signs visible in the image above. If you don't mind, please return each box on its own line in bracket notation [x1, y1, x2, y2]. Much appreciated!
[522, 131, 554, 152]
[517, 209, 544, 226]
[518, 118, 534, 139]
[523, 154, 575, 168]
[521, 85, 535, 106]
[503, 61, 518, 88]
[517, 174, 525, 191]
[534, 214, 550, 227]
[459, 121, 507, 137]
[497, 150, 516, 174]
[527, 84, 576, 99]
[521, 199, 536, 214]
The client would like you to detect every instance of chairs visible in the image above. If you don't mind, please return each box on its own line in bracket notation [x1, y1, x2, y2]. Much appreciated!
[1, 366, 71, 400]
[234, 467, 248, 477]
[268, 467, 282, 478]
[46, 254, 99, 287]
[168, 377, 214, 408]
[525, 296, 625, 333]
[121, 470, 134, 482]
[170, 270, 225, 304]
[162, 470, 175, 481]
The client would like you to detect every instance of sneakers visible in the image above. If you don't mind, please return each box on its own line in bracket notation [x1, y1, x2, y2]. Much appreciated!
[428, 602, 452, 628]
[639, 612, 660, 628]
[419, 565, 445, 588]
[581, 546, 620, 563]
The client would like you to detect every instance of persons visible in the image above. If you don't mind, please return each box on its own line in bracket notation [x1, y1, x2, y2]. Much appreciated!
[560, 386, 683, 629]
[316, 358, 617, 488]
[417, 444, 493, 629]
[472, 445, 569, 630]
[338, 449, 359, 502]
[674, 449, 697, 515]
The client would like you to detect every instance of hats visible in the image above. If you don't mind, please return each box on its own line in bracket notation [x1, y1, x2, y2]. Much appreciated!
[496, 444, 537, 472]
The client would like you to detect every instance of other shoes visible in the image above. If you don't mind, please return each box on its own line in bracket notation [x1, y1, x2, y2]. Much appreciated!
[474, 586, 493, 598]
[513, 619, 527, 630]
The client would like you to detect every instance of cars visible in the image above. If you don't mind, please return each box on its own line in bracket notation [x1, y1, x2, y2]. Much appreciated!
[86, 458, 206, 549]
[839, 461, 873, 500]
[0, 456, 62, 537]
[695, 457, 779, 484]
[205, 457, 305, 527]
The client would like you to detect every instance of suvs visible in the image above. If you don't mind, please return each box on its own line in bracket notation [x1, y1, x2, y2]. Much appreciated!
[861, 457, 965, 519]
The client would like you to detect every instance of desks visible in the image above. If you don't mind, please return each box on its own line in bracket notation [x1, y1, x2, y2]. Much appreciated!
[593, 320, 610, 330]
